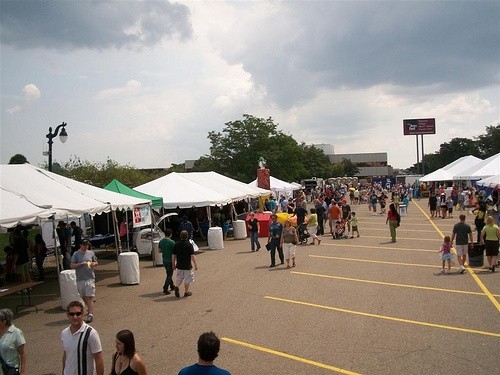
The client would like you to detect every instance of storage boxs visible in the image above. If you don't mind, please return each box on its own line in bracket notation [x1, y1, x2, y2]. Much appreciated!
[245, 212, 271, 238]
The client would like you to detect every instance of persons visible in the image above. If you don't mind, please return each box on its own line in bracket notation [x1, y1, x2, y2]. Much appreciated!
[158, 230, 177, 294]
[0, 308, 27, 375]
[280, 219, 299, 268]
[451, 214, 473, 273]
[247, 212, 261, 252]
[440, 236, 453, 273]
[70, 239, 98, 323]
[268, 215, 284, 267]
[172, 231, 198, 297]
[110, 330, 149, 375]
[178, 332, 231, 375]
[61, 301, 104, 375]
[0, 183, 500, 294]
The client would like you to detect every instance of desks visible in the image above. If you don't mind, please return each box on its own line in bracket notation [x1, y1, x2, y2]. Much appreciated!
[88, 234, 114, 252]
[399, 205, 407, 216]
[421, 191, 431, 199]
[0, 281, 44, 314]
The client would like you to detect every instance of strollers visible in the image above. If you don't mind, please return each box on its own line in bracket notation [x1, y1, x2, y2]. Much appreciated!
[294, 222, 311, 245]
[331, 218, 349, 240]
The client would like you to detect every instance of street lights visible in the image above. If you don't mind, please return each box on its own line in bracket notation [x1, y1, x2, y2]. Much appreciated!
[46, 122, 69, 172]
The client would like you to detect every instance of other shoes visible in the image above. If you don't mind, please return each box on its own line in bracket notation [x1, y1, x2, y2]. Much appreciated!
[184, 292, 192, 297]
[310, 243, 314, 245]
[256, 246, 260, 251]
[270, 263, 275, 267]
[441, 269, 451, 274]
[171, 286, 175, 291]
[489, 264, 495, 272]
[87, 314, 93, 322]
[175, 287, 179, 297]
[318, 239, 321, 245]
[163, 290, 171, 294]
[22, 287, 32, 292]
[431, 215, 453, 219]
[460, 265, 465, 274]
[281, 259, 284, 264]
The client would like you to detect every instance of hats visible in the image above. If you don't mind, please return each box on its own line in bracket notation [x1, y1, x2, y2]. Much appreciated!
[441, 193, 445, 195]
[342, 199, 346, 203]
[331, 199, 335, 202]
[80, 239, 89, 245]
[57, 221, 66, 227]
[180, 216, 188, 221]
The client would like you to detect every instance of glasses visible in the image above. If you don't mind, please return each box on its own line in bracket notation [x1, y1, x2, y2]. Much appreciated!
[69, 312, 82, 316]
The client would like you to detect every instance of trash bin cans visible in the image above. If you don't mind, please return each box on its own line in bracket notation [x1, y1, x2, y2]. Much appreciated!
[399, 205, 407, 216]
[468, 243, 486, 266]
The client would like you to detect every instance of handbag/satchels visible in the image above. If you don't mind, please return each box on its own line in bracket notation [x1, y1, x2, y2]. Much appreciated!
[266, 238, 271, 251]
[2, 365, 20, 375]
[189, 239, 199, 251]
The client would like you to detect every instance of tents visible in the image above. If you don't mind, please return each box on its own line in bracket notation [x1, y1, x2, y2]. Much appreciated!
[0, 163, 303, 275]
[419, 153, 500, 189]
